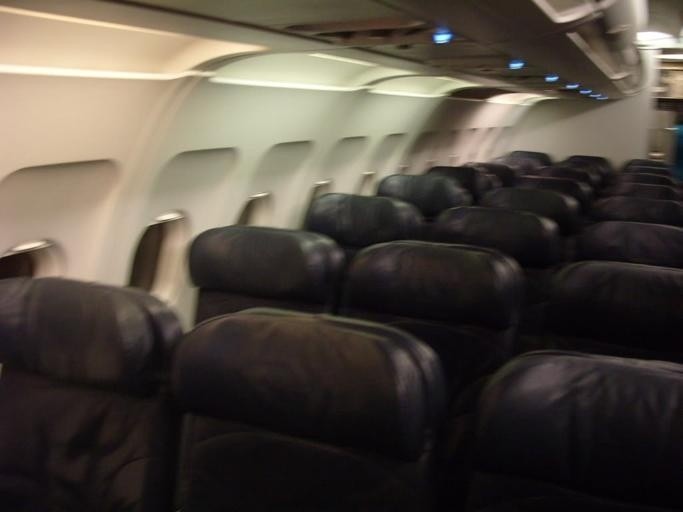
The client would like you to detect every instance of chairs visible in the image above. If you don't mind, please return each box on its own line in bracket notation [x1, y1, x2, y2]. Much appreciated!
[0, 149, 682, 511]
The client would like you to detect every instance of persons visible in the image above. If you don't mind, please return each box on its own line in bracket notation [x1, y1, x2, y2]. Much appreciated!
[671, 115, 683, 164]
